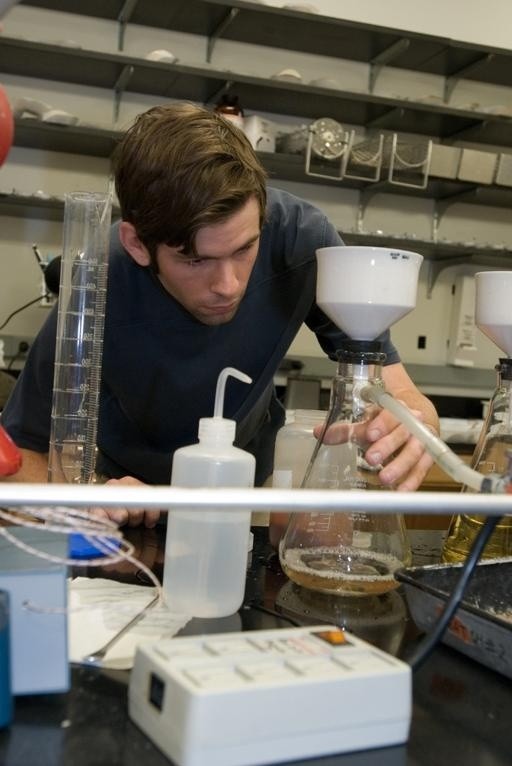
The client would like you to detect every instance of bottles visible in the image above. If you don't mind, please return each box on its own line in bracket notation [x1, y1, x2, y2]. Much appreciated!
[161, 365, 257, 620]
[277, 339, 413, 599]
[439, 356, 511, 566]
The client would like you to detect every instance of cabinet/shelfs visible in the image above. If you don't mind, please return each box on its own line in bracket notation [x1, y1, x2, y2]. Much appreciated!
[1, 0, 512, 299]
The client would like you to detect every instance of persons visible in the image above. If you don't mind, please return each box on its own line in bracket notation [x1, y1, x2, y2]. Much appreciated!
[0, 104, 441, 532]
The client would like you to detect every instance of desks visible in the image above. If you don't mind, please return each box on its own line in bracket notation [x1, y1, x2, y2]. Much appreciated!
[0, 528, 511, 766]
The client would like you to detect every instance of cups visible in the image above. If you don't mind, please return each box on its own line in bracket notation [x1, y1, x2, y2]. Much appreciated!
[47, 190, 113, 484]
